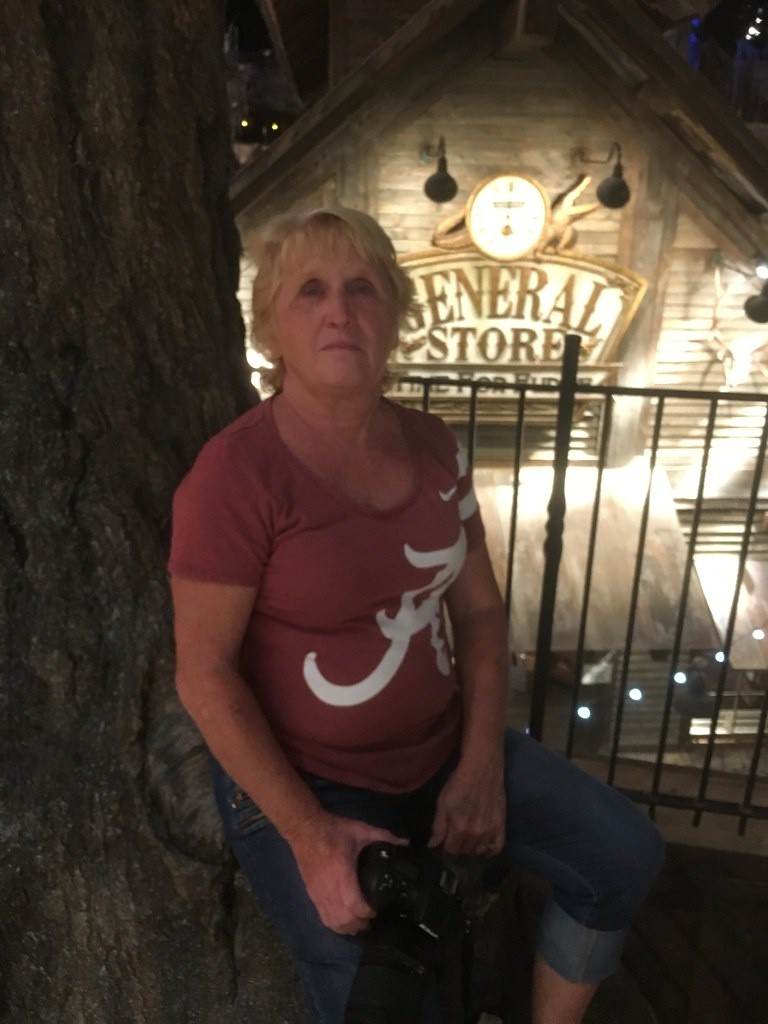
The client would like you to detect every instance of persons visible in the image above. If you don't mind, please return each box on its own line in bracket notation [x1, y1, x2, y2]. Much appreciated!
[168, 207, 664, 1024]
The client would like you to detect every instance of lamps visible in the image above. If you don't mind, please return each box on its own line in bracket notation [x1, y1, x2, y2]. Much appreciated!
[419, 134, 458, 202]
[573, 142, 631, 208]
[707, 254, 768, 322]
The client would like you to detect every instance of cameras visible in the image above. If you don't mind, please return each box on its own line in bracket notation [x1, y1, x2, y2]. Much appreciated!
[359, 841, 466, 961]
